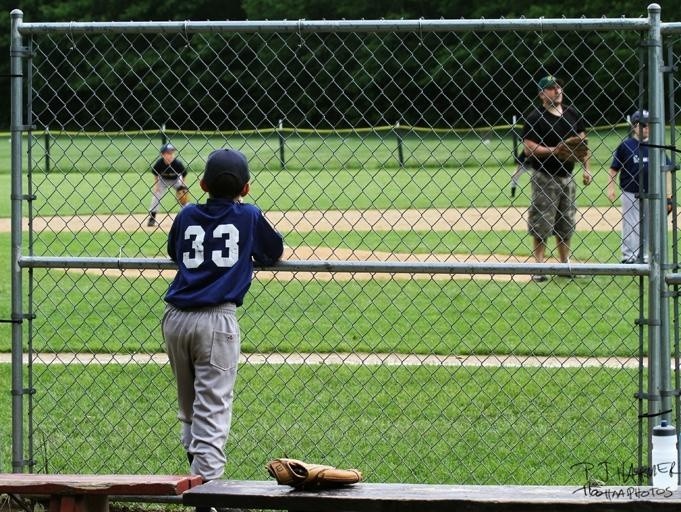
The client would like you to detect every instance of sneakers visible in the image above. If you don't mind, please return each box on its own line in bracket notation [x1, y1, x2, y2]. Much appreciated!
[534, 275, 546, 282]
[148, 218, 156, 226]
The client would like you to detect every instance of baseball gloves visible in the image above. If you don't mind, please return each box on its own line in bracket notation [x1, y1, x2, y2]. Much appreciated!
[266, 458, 361, 486]
[557, 137, 589, 161]
[176, 186, 189, 204]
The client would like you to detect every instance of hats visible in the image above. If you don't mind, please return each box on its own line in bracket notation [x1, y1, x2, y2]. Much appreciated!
[537, 75, 565, 91]
[630, 108, 650, 124]
[161, 143, 176, 154]
[203, 148, 250, 195]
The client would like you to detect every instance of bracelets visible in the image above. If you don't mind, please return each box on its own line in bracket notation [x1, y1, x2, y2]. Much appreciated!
[668, 198, 673, 205]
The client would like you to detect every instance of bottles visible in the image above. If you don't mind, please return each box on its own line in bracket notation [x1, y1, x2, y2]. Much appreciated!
[652, 420, 679, 491]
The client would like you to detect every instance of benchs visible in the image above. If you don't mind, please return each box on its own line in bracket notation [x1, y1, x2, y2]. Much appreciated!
[1, 472, 681, 511]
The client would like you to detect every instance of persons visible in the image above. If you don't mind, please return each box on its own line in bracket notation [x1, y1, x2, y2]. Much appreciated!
[159, 147, 284, 510]
[508, 151, 533, 197]
[607, 109, 675, 264]
[521, 74, 593, 284]
[147, 144, 191, 227]
